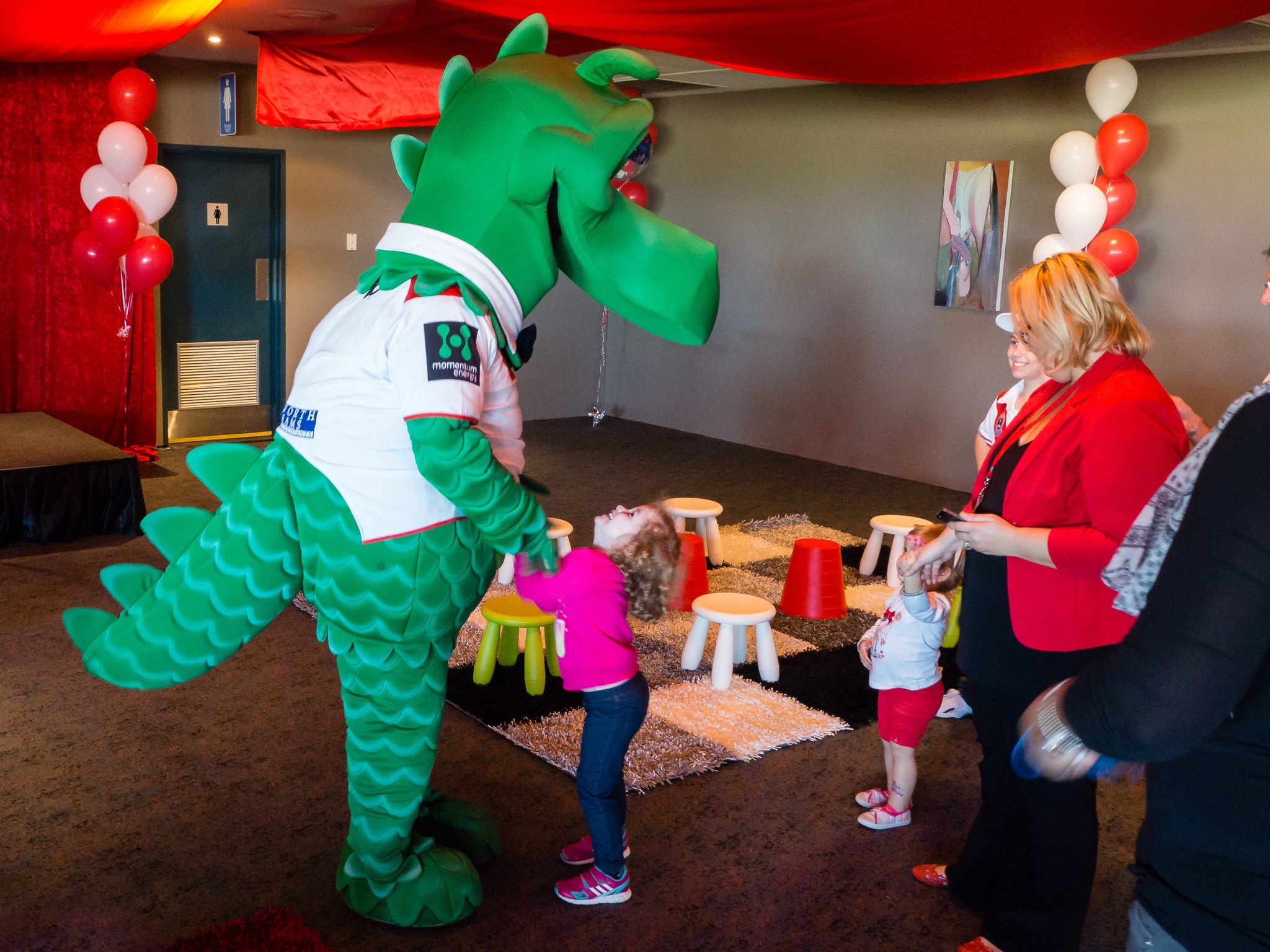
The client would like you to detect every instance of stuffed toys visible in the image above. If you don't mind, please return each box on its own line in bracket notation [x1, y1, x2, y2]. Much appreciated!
[59, 13, 719, 929]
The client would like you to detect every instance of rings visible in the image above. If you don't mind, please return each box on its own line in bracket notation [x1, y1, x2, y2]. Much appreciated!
[964, 542, 971, 551]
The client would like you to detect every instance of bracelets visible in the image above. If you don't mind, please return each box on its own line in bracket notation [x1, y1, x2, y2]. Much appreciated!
[1188, 416, 1202, 437]
[1037, 677, 1088, 758]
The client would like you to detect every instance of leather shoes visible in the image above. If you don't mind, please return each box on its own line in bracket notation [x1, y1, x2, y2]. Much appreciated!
[911, 864, 950, 889]
[957, 937, 995, 952]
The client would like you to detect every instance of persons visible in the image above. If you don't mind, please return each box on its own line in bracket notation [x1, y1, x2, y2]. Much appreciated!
[973, 320, 1051, 476]
[897, 250, 1189, 952]
[1017, 255, 1270, 950]
[852, 522, 966, 830]
[512, 504, 686, 905]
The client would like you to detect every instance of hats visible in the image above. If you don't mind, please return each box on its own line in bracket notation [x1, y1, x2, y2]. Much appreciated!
[994, 312, 1020, 333]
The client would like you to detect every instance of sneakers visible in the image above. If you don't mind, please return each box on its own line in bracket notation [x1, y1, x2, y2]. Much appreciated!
[554, 864, 632, 905]
[560, 828, 631, 866]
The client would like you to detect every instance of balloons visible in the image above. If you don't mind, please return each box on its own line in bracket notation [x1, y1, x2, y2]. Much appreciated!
[71, 68, 178, 293]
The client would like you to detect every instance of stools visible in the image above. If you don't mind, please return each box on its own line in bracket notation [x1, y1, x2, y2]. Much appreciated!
[497, 518, 573, 585]
[669, 533, 710, 611]
[778, 538, 848, 618]
[473, 593, 561, 696]
[662, 497, 723, 567]
[860, 514, 936, 586]
[680, 592, 779, 689]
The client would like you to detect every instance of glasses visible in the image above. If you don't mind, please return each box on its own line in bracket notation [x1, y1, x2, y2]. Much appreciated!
[1015, 324, 1032, 345]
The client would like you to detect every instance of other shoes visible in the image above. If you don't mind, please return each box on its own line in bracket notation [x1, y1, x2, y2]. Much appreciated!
[857, 805, 912, 830]
[855, 788, 913, 808]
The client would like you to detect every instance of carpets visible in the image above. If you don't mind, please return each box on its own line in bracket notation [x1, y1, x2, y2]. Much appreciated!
[441, 510, 873, 795]
